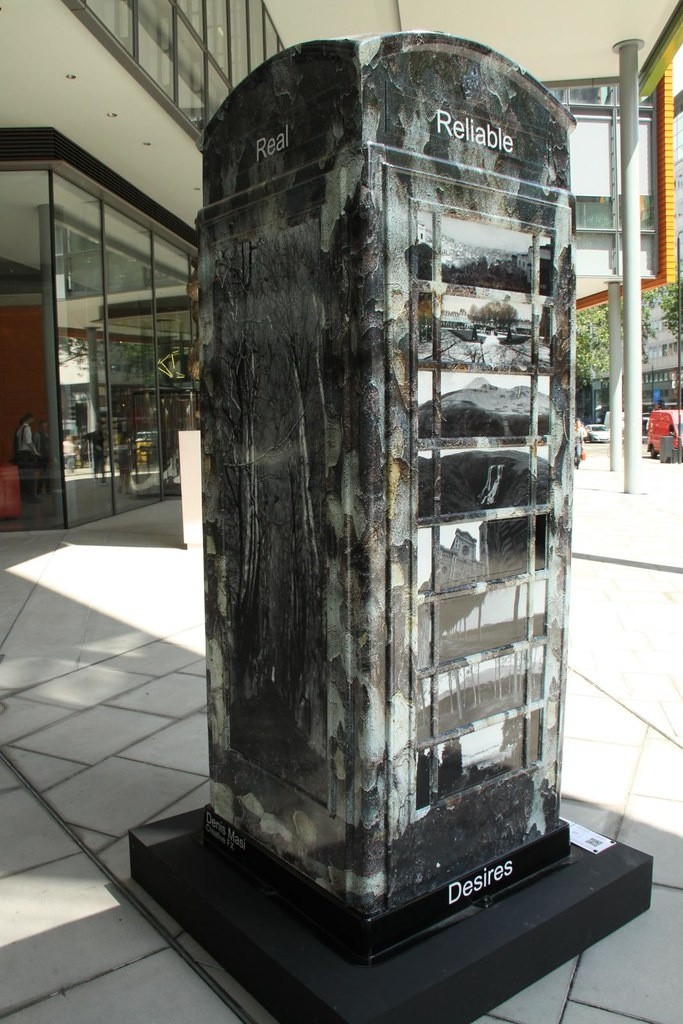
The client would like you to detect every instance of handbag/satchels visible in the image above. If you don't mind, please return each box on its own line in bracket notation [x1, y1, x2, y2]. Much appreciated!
[580, 452, 586, 460]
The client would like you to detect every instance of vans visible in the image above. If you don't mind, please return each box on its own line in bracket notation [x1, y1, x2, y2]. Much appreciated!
[646, 409, 683, 459]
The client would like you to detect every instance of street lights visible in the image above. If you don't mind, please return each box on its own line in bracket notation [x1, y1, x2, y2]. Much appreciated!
[57, 353, 117, 374]
[583, 320, 596, 423]
[642, 362, 653, 411]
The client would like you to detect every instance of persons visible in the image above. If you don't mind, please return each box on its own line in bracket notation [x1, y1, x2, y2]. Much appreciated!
[33, 422, 51, 495]
[115, 424, 130, 494]
[15, 415, 42, 504]
[63, 436, 76, 475]
[81, 424, 104, 479]
[575, 418, 588, 470]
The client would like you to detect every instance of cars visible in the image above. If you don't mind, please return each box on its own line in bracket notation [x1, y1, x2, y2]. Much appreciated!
[585, 423, 611, 442]
[604, 411, 650, 443]
[581, 422, 587, 442]
[112, 431, 158, 467]
